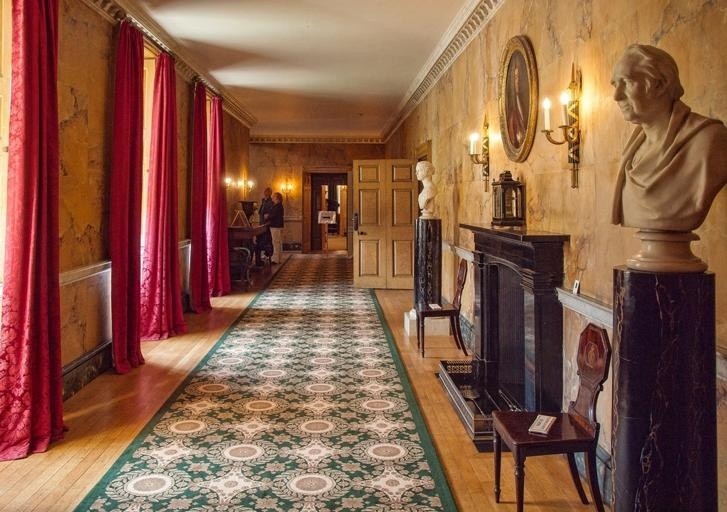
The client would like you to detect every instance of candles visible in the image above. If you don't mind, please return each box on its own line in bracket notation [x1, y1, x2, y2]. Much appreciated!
[469, 132, 479, 154]
[541, 92, 571, 131]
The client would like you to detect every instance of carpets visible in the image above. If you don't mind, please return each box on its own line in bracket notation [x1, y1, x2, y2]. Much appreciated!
[69, 253, 466, 511]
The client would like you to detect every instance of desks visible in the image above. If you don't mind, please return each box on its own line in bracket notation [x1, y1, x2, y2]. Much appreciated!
[227, 222, 275, 291]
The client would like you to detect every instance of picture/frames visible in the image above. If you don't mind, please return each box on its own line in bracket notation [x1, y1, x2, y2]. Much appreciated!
[497, 34, 541, 164]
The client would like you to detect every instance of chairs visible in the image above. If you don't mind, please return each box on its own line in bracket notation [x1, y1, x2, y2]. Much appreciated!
[416, 258, 469, 360]
[489, 323, 611, 512]
[228, 247, 252, 294]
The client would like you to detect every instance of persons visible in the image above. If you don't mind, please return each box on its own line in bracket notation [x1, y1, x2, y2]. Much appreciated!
[257, 186, 273, 259]
[264, 191, 285, 264]
[413, 159, 437, 210]
[606, 43, 725, 233]
[508, 66, 527, 149]
